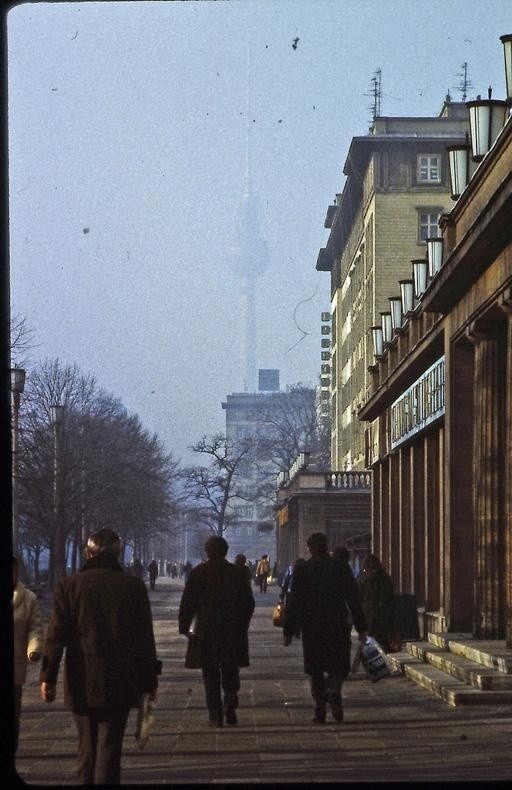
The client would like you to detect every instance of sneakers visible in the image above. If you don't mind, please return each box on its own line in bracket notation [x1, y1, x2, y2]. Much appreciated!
[207, 720, 222, 727]
[312, 716, 326, 723]
[325, 692, 343, 721]
[223, 702, 237, 725]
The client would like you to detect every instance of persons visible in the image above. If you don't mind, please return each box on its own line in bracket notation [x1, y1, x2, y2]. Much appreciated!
[40, 528, 159, 786]
[178, 536, 255, 727]
[12, 557, 45, 786]
[279, 531, 395, 723]
[146, 553, 269, 593]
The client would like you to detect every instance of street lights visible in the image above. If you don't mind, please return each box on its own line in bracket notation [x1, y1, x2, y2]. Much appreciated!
[11, 361, 25, 560]
[49, 400, 65, 521]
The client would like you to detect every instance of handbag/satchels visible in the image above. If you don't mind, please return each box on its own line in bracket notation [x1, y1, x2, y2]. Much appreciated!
[385, 619, 401, 653]
[273, 608, 284, 627]
[360, 637, 389, 682]
